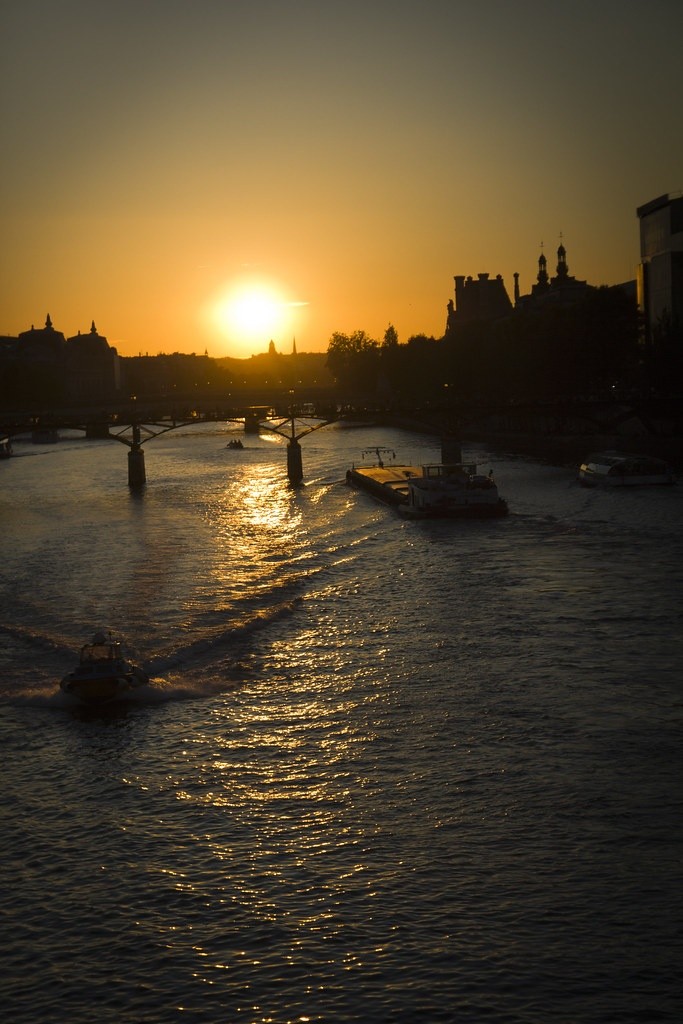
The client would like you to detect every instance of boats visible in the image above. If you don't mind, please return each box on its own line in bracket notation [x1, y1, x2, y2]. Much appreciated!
[59, 632, 149, 704]
[579, 452, 678, 487]
[227, 443, 243, 449]
[346, 447, 509, 520]
